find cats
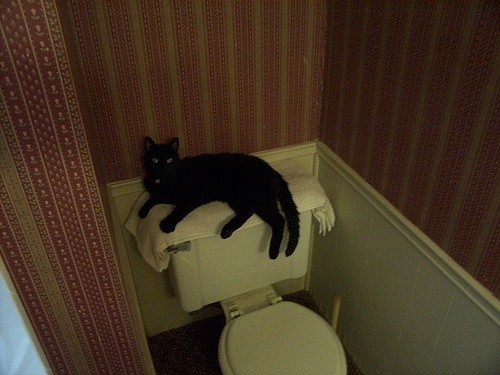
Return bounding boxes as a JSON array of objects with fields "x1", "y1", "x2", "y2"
[{"x1": 138, "y1": 136, "x2": 301, "y2": 260}]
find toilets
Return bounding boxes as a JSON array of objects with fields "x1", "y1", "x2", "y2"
[{"x1": 167, "y1": 160, "x2": 347, "y2": 374}]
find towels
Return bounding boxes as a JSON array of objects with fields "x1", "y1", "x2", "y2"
[{"x1": 123, "y1": 158, "x2": 335, "y2": 272}]
